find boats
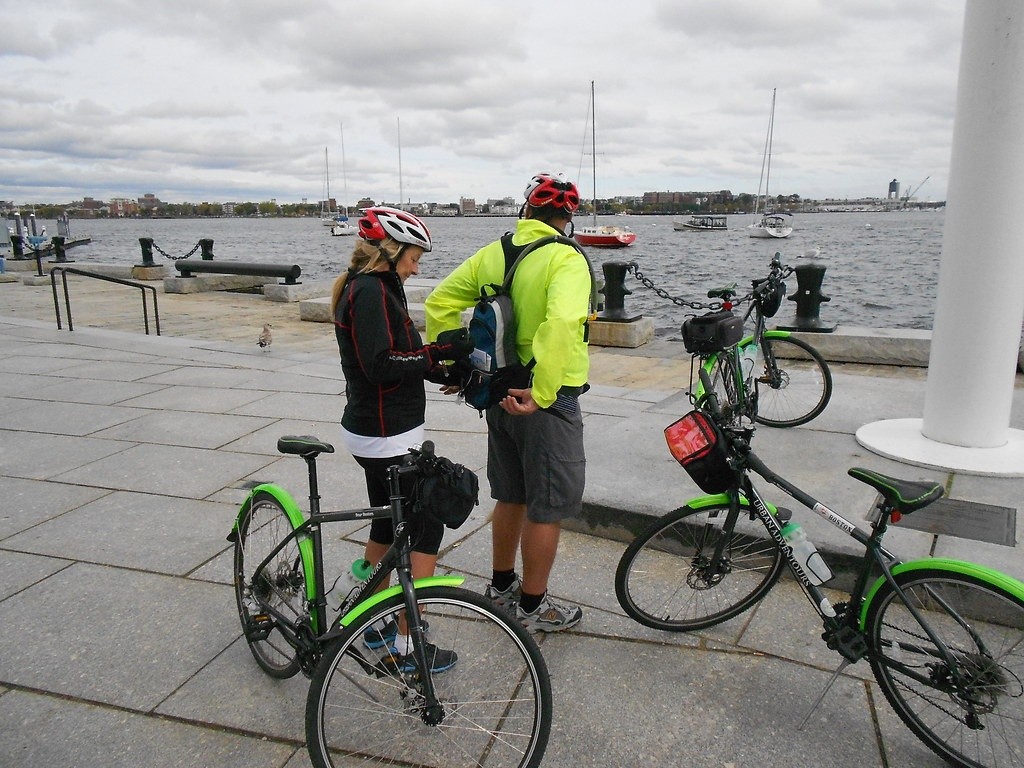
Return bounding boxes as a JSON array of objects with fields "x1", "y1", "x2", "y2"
[{"x1": 672, "y1": 214, "x2": 729, "y2": 232}]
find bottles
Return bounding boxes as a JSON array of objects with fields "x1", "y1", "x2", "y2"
[
  {"x1": 738, "y1": 346, "x2": 748, "y2": 381},
  {"x1": 781, "y1": 522, "x2": 832, "y2": 585},
  {"x1": 326, "y1": 559, "x2": 374, "y2": 610},
  {"x1": 745, "y1": 345, "x2": 758, "y2": 378}
]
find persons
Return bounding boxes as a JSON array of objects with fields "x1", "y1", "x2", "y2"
[
  {"x1": 424, "y1": 168, "x2": 591, "y2": 633},
  {"x1": 332, "y1": 206, "x2": 469, "y2": 673}
]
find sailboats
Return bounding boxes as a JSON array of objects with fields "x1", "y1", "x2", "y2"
[
  {"x1": 746, "y1": 86, "x2": 797, "y2": 239},
  {"x1": 570, "y1": 79, "x2": 637, "y2": 248},
  {"x1": 328, "y1": 121, "x2": 360, "y2": 237},
  {"x1": 320, "y1": 146, "x2": 341, "y2": 226}
]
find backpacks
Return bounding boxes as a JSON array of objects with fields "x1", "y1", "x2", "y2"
[{"x1": 459, "y1": 233, "x2": 597, "y2": 410}]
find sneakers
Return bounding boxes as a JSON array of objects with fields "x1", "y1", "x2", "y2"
[
  {"x1": 484, "y1": 574, "x2": 522, "y2": 608},
  {"x1": 389, "y1": 639, "x2": 457, "y2": 672},
  {"x1": 364, "y1": 612, "x2": 429, "y2": 648},
  {"x1": 515, "y1": 592, "x2": 582, "y2": 634}
]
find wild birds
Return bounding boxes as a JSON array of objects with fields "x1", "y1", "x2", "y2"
[
  {"x1": 254, "y1": 323, "x2": 274, "y2": 353},
  {"x1": 796, "y1": 247, "x2": 821, "y2": 264}
]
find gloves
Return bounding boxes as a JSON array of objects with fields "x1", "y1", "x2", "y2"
[
  {"x1": 440, "y1": 360, "x2": 472, "y2": 386},
  {"x1": 436, "y1": 328, "x2": 475, "y2": 360}
]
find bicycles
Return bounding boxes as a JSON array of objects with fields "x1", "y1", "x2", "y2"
[
  {"x1": 678, "y1": 251, "x2": 834, "y2": 429},
  {"x1": 226, "y1": 434, "x2": 556, "y2": 768},
  {"x1": 615, "y1": 364, "x2": 1024, "y2": 768}
]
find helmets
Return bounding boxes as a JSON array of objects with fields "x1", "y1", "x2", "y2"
[
  {"x1": 359, "y1": 206, "x2": 432, "y2": 253},
  {"x1": 525, "y1": 172, "x2": 580, "y2": 213}
]
